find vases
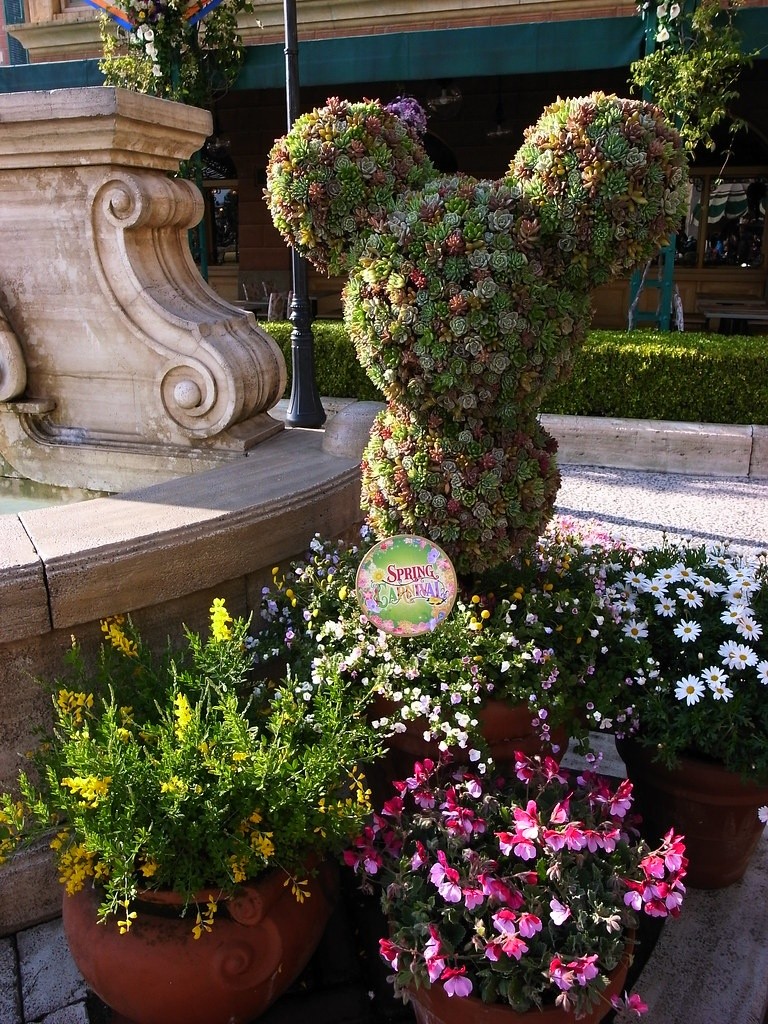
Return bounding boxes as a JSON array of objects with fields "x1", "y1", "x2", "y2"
[
  {"x1": 59, "y1": 847, "x2": 337, "y2": 1024},
  {"x1": 387, "y1": 913, "x2": 634, "y2": 1024},
  {"x1": 363, "y1": 691, "x2": 574, "y2": 780},
  {"x1": 641, "y1": 740, "x2": 768, "y2": 890}
]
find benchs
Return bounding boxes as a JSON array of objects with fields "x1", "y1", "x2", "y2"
[{"x1": 694, "y1": 305, "x2": 768, "y2": 337}]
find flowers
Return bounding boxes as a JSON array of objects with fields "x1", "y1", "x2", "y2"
[
  {"x1": 578, "y1": 527, "x2": 768, "y2": 829},
  {"x1": 252, "y1": 522, "x2": 584, "y2": 755},
  {"x1": 0, "y1": 595, "x2": 394, "y2": 941},
  {"x1": 336, "y1": 727, "x2": 691, "y2": 1018}
]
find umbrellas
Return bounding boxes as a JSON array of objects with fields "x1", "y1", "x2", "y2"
[{"x1": 692, "y1": 182, "x2": 749, "y2": 224}]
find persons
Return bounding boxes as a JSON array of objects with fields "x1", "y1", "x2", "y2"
[
  {"x1": 676, "y1": 224, "x2": 697, "y2": 254},
  {"x1": 707, "y1": 232, "x2": 737, "y2": 261},
  {"x1": 751, "y1": 234, "x2": 760, "y2": 256}
]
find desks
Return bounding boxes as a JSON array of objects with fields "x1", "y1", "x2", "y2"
[{"x1": 698, "y1": 295, "x2": 765, "y2": 333}]
